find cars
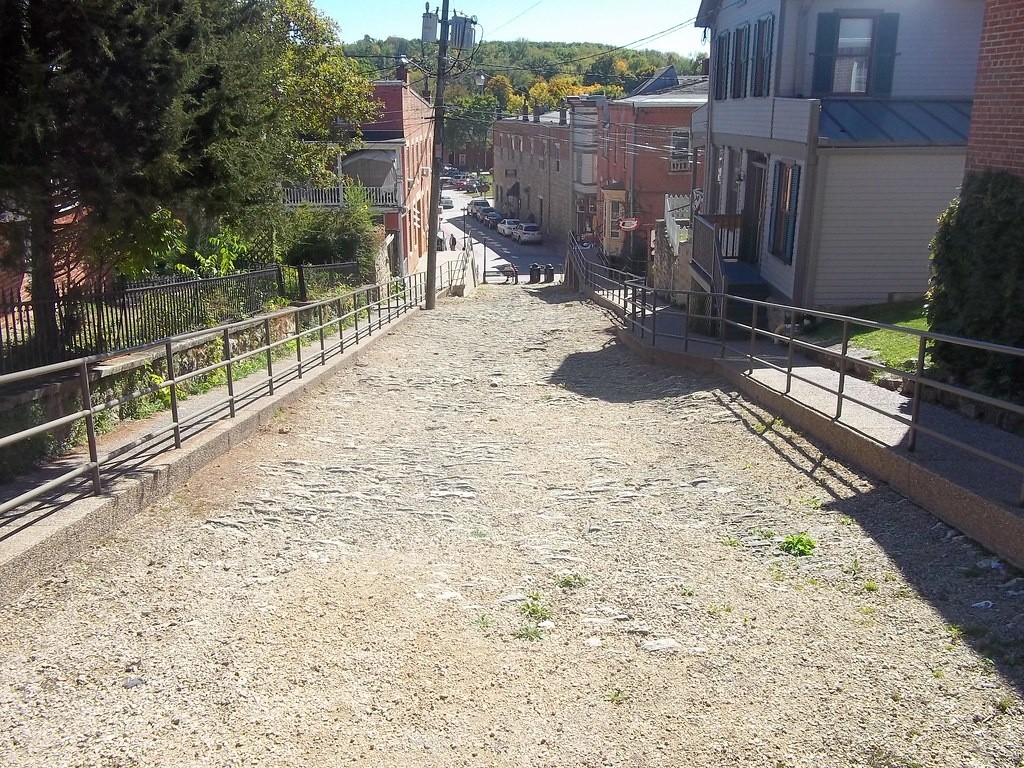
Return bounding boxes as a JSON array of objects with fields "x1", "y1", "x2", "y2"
[
  {"x1": 439, "y1": 195, "x2": 454, "y2": 209},
  {"x1": 453, "y1": 175, "x2": 469, "y2": 184},
  {"x1": 397, "y1": 53, "x2": 486, "y2": 311},
  {"x1": 484, "y1": 212, "x2": 502, "y2": 229},
  {"x1": 511, "y1": 223, "x2": 543, "y2": 245},
  {"x1": 476, "y1": 206, "x2": 495, "y2": 222},
  {"x1": 497, "y1": 219, "x2": 522, "y2": 238},
  {"x1": 466, "y1": 199, "x2": 490, "y2": 217}
]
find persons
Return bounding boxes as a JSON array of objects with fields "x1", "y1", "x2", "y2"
[
  {"x1": 527, "y1": 213, "x2": 534, "y2": 223},
  {"x1": 504, "y1": 263, "x2": 514, "y2": 282},
  {"x1": 503, "y1": 203, "x2": 507, "y2": 213},
  {"x1": 449, "y1": 234, "x2": 456, "y2": 251}
]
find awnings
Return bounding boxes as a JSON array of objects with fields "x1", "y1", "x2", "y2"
[{"x1": 506, "y1": 182, "x2": 519, "y2": 197}]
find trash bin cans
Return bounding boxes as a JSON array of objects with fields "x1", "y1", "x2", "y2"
[
  {"x1": 544, "y1": 263, "x2": 554, "y2": 282},
  {"x1": 529, "y1": 262, "x2": 541, "y2": 282}
]
